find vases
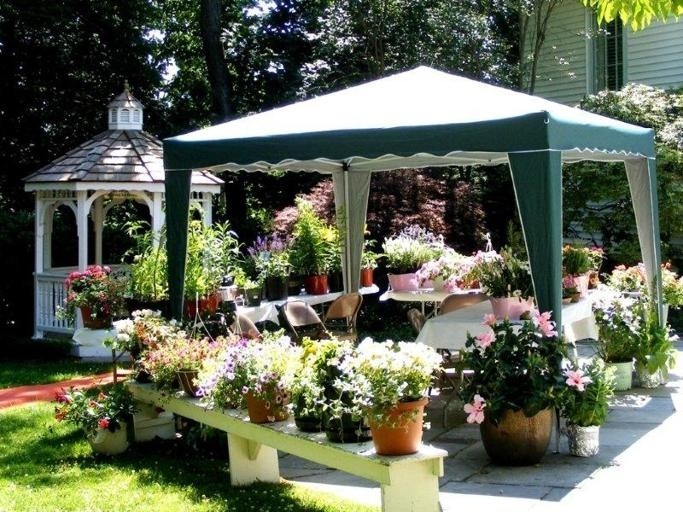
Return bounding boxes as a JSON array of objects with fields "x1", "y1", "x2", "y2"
[
  {"x1": 82, "y1": 419, "x2": 128, "y2": 456},
  {"x1": 478, "y1": 398, "x2": 599, "y2": 465},
  {"x1": 365, "y1": 392, "x2": 429, "y2": 455}
]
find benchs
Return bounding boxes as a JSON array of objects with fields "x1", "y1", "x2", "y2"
[{"x1": 122, "y1": 379, "x2": 446, "y2": 511}]
